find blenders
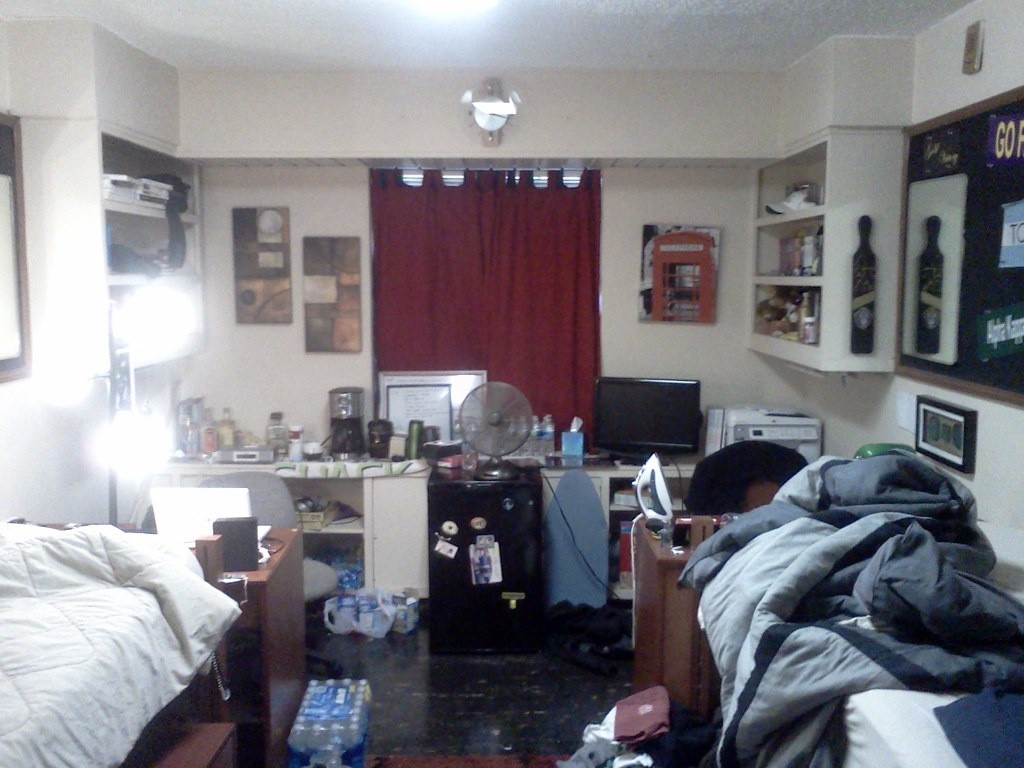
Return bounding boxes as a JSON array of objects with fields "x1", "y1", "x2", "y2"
[{"x1": 329, "y1": 386, "x2": 366, "y2": 460}]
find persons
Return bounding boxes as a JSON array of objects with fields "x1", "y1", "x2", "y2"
[{"x1": 472, "y1": 549, "x2": 492, "y2": 584}]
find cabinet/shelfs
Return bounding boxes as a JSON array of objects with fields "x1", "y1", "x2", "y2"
[
  {"x1": 747, "y1": 127, "x2": 905, "y2": 377},
  {"x1": 103, "y1": 139, "x2": 207, "y2": 359},
  {"x1": 292, "y1": 466, "x2": 429, "y2": 603},
  {"x1": 543, "y1": 477, "x2": 728, "y2": 603}
]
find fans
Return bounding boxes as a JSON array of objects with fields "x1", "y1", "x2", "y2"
[{"x1": 458, "y1": 379, "x2": 533, "y2": 476}]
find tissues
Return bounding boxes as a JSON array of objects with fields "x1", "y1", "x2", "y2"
[{"x1": 561, "y1": 415, "x2": 585, "y2": 456}]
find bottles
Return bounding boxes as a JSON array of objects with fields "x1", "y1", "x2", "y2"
[
  {"x1": 265, "y1": 412, "x2": 289, "y2": 461},
  {"x1": 198, "y1": 407, "x2": 218, "y2": 453},
  {"x1": 461, "y1": 415, "x2": 555, "y2": 470},
  {"x1": 217, "y1": 408, "x2": 237, "y2": 450},
  {"x1": 288, "y1": 677, "x2": 373, "y2": 768}
]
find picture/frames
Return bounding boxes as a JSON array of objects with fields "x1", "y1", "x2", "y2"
[
  {"x1": 386, "y1": 383, "x2": 451, "y2": 445},
  {"x1": 378, "y1": 369, "x2": 490, "y2": 429},
  {"x1": 909, "y1": 396, "x2": 978, "y2": 472}
]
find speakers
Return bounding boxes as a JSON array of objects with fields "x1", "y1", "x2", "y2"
[{"x1": 213, "y1": 518, "x2": 259, "y2": 572}]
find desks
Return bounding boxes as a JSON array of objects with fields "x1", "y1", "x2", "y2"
[
  {"x1": 46, "y1": 525, "x2": 307, "y2": 768},
  {"x1": 425, "y1": 464, "x2": 543, "y2": 652},
  {"x1": 630, "y1": 513, "x2": 724, "y2": 728}
]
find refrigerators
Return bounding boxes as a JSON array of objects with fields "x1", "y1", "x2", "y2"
[{"x1": 427, "y1": 464, "x2": 547, "y2": 655}]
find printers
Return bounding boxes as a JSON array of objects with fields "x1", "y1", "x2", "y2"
[{"x1": 705, "y1": 406, "x2": 822, "y2": 467}]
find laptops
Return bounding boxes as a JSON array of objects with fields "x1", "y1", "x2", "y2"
[{"x1": 148, "y1": 484, "x2": 271, "y2": 549}]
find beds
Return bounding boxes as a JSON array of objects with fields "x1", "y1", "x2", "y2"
[
  {"x1": 675, "y1": 477, "x2": 1024, "y2": 768},
  {"x1": 0, "y1": 513, "x2": 249, "y2": 768}
]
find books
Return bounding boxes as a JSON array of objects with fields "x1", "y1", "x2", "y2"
[{"x1": 103, "y1": 173, "x2": 174, "y2": 210}]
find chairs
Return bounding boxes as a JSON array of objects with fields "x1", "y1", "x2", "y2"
[
  {"x1": 685, "y1": 440, "x2": 810, "y2": 520},
  {"x1": 201, "y1": 470, "x2": 344, "y2": 680}
]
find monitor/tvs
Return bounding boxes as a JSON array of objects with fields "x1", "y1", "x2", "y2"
[{"x1": 592, "y1": 376, "x2": 700, "y2": 466}]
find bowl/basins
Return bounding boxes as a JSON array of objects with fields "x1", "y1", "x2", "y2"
[{"x1": 303, "y1": 441, "x2": 327, "y2": 459}]
